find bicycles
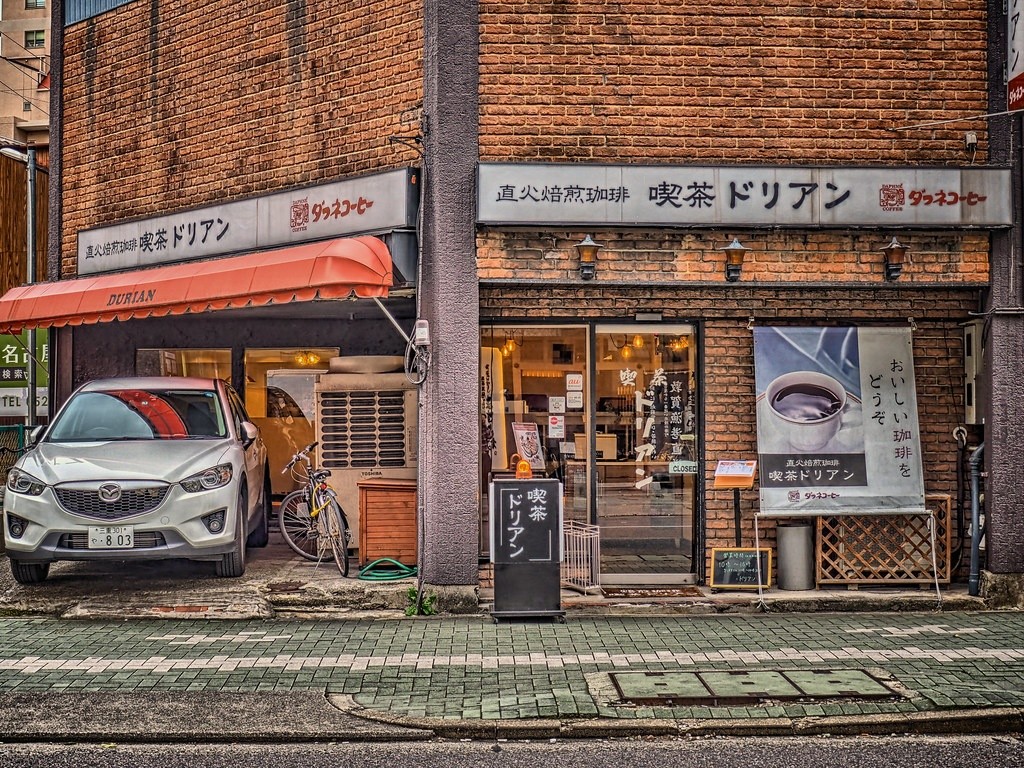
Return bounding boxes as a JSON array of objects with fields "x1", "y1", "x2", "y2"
[{"x1": 278, "y1": 441, "x2": 352, "y2": 578}]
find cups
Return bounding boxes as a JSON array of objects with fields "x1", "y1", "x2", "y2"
[{"x1": 765, "y1": 371, "x2": 848, "y2": 452}]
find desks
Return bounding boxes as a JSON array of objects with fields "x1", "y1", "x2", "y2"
[
  {"x1": 566, "y1": 461, "x2": 684, "y2": 518},
  {"x1": 523, "y1": 412, "x2": 637, "y2": 461}
]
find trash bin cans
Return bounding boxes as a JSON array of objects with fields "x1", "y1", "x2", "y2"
[{"x1": 777, "y1": 522, "x2": 814, "y2": 591}]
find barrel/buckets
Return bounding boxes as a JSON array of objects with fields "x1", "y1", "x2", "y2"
[{"x1": 775, "y1": 525, "x2": 815, "y2": 591}]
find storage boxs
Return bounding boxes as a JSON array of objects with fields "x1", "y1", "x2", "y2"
[
  {"x1": 622, "y1": 334, "x2": 632, "y2": 358},
  {"x1": 574, "y1": 432, "x2": 618, "y2": 460},
  {"x1": 633, "y1": 333, "x2": 644, "y2": 348}
]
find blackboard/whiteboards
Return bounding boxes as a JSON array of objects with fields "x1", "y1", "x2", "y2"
[
  {"x1": 491, "y1": 479, "x2": 566, "y2": 623},
  {"x1": 710, "y1": 548, "x2": 772, "y2": 588}
]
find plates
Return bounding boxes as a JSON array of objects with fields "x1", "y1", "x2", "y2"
[{"x1": 756, "y1": 387, "x2": 862, "y2": 453}]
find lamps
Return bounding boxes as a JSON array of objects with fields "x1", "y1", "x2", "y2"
[
  {"x1": 295, "y1": 350, "x2": 321, "y2": 367},
  {"x1": 879, "y1": 236, "x2": 911, "y2": 282},
  {"x1": 501, "y1": 328, "x2": 516, "y2": 356},
  {"x1": 572, "y1": 234, "x2": 604, "y2": 281},
  {"x1": 720, "y1": 235, "x2": 752, "y2": 283}
]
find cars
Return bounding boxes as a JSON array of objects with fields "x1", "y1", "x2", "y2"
[{"x1": 2, "y1": 376, "x2": 272, "y2": 585}]
